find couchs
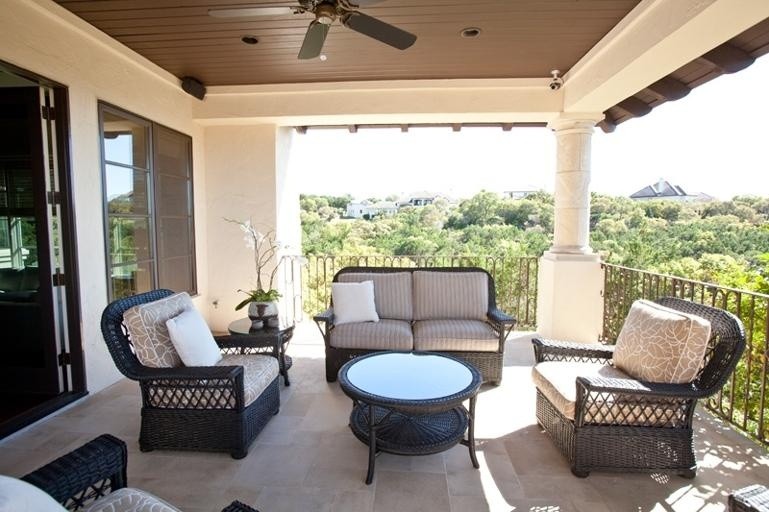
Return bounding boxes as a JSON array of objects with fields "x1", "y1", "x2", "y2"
[
  {"x1": 312, "y1": 266, "x2": 517, "y2": 387},
  {"x1": 531, "y1": 296, "x2": 748, "y2": 480}
]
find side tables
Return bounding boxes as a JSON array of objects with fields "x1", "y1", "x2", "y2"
[{"x1": 228, "y1": 315, "x2": 296, "y2": 386}]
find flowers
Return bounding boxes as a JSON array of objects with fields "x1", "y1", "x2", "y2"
[{"x1": 220, "y1": 217, "x2": 299, "y2": 311}]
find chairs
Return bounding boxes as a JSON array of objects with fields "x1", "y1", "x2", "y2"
[
  {"x1": 0, "y1": 434, "x2": 260, "y2": 512},
  {"x1": 99, "y1": 288, "x2": 281, "y2": 459}
]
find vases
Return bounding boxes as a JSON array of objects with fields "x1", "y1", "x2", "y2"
[{"x1": 247, "y1": 302, "x2": 277, "y2": 321}]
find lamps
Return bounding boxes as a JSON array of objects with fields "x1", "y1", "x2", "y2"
[{"x1": 315, "y1": 3, "x2": 335, "y2": 25}]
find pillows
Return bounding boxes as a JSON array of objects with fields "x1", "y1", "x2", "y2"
[
  {"x1": 1, "y1": 475, "x2": 68, "y2": 512},
  {"x1": 165, "y1": 308, "x2": 223, "y2": 385},
  {"x1": 329, "y1": 280, "x2": 379, "y2": 328}
]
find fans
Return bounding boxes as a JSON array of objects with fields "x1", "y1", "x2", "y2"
[{"x1": 209, "y1": 0, "x2": 418, "y2": 61}]
